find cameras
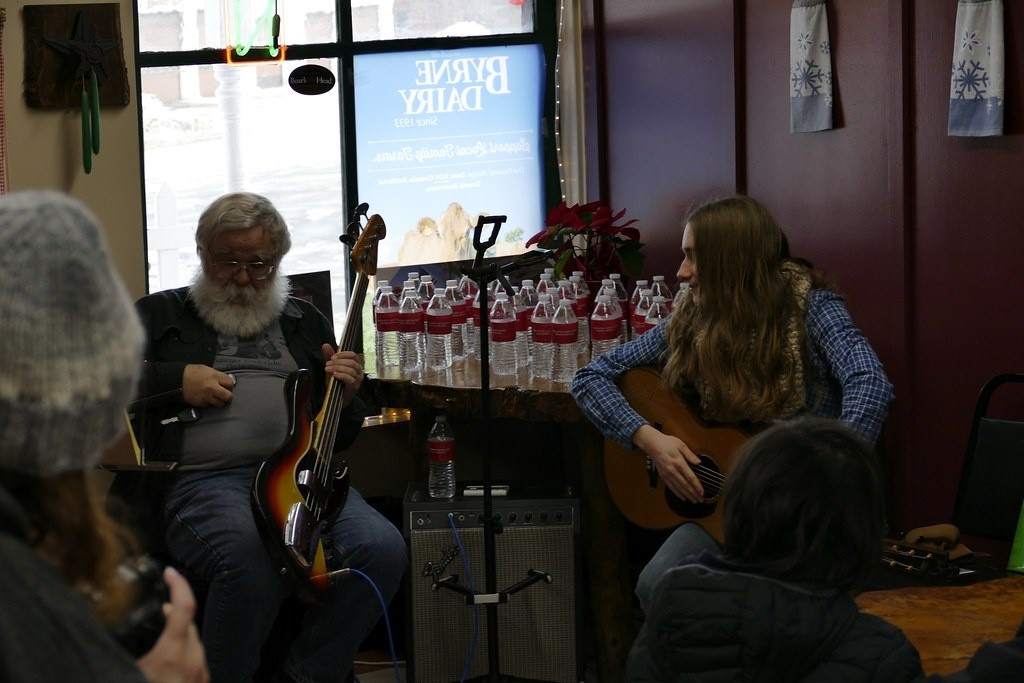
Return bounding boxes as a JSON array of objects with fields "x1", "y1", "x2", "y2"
[{"x1": 78, "y1": 553, "x2": 172, "y2": 661}]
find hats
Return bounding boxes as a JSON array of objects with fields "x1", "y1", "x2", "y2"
[{"x1": 2, "y1": 187, "x2": 148, "y2": 480}]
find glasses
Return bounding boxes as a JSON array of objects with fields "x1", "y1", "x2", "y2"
[{"x1": 205, "y1": 247, "x2": 279, "y2": 283}]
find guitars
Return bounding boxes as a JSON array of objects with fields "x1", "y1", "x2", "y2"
[
  {"x1": 604, "y1": 367, "x2": 1000, "y2": 588},
  {"x1": 254, "y1": 202, "x2": 387, "y2": 609}
]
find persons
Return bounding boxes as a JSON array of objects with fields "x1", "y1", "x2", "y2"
[
  {"x1": 570, "y1": 197, "x2": 895, "y2": 616},
  {"x1": 622, "y1": 421, "x2": 1023, "y2": 683},
  {"x1": 130, "y1": 193, "x2": 407, "y2": 683},
  {"x1": 1, "y1": 189, "x2": 207, "y2": 683}
]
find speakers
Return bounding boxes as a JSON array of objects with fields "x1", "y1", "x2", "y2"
[{"x1": 404, "y1": 412, "x2": 580, "y2": 683}]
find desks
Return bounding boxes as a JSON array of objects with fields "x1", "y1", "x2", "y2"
[{"x1": 347, "y1": 351, "x2": 632, "y2": 683}]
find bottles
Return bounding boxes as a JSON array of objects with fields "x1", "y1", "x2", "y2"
[
  {"x1": 425, "y1": 415, "x2": 456, "y2": 501},
  {"x1": 373, "y1": 266, "x2": 689, "y2": 385}
]
